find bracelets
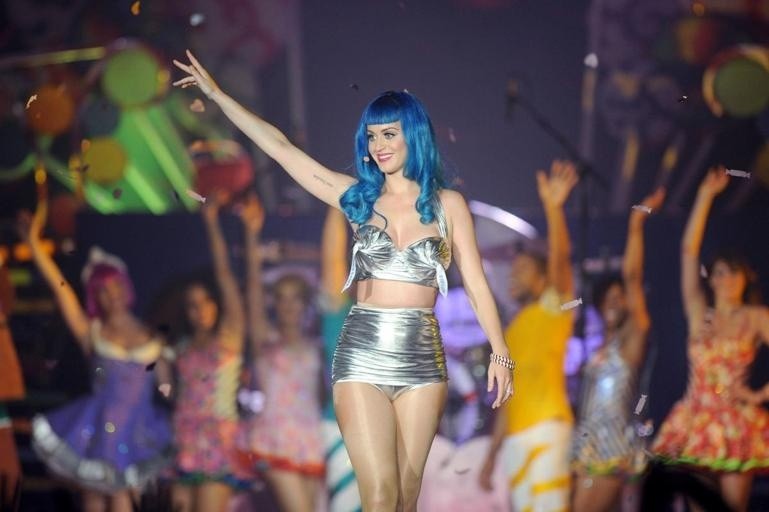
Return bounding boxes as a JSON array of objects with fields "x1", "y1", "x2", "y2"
[{"x1": 489, "y1": 353, "x2": 514, "y2": 371}]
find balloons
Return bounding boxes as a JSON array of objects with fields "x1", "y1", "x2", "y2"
[
  {"x1": 27, "y1": 88, "x2": 74, "y2": 134},
  {"x1": 85, "y1": 141, "x2": 126, "y2": 186},
  {"x1": 702, "y1": 53, "x2": 768, "y2": 113},
  {"x1": 99, "y1": 49, "x2": 159, "y2": 104}
]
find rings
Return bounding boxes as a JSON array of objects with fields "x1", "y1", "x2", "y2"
[{"x1": 506, "y1": 391, "x2": 513, "y2": 394}]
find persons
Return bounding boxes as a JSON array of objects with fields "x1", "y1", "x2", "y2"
[
  {"x1": 655, "y1": 164, "x2": 769, "y2": 512},
  {"x1": 573, "y1": 181, "x2": 669, "y2": 512},
  {"x1": 235, "y1": 193, "x2": 332, "y2": 512},
  {"x1": 173, "y1": 49, "x2": 514, "y2": 512},
  {"x1": 30, "y1": 165, "x2": 168, "y2": 511},
  {"x1": 170, "y1": 184, "x2": 246, "y2": 510},
  {"x1": 499, "y1": 153, "x2": 580, "y2": 511}
]
